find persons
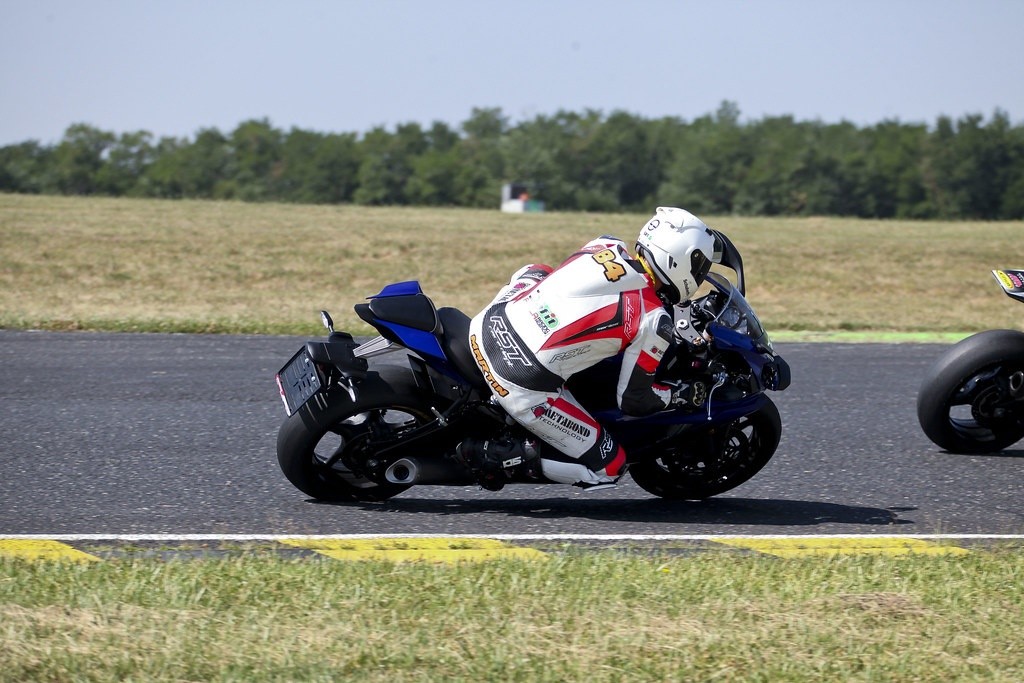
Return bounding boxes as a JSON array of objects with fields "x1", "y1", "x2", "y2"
[{"x1": 456, "y1": 207, "x2": 712, "y2": 491}]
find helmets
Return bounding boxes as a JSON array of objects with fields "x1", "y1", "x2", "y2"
[{"x1": 635, "y1": 206, "x2": 716, "y2": 307}]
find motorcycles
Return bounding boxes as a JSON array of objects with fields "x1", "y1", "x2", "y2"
[
  {"x1": 274, "y1": 231, "x2": 793, "y2": 507},
  {"x1": 916, "y1": 269, "x2": 1024, "y2": 455}
]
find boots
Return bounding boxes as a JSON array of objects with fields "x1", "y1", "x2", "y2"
[{"x1": 456, "y1": 437, "x2": 549, "y2": 491}]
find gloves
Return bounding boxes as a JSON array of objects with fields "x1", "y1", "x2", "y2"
[{"x1": 661, "y1": 378, "x2": 707, "y2": 412}]
